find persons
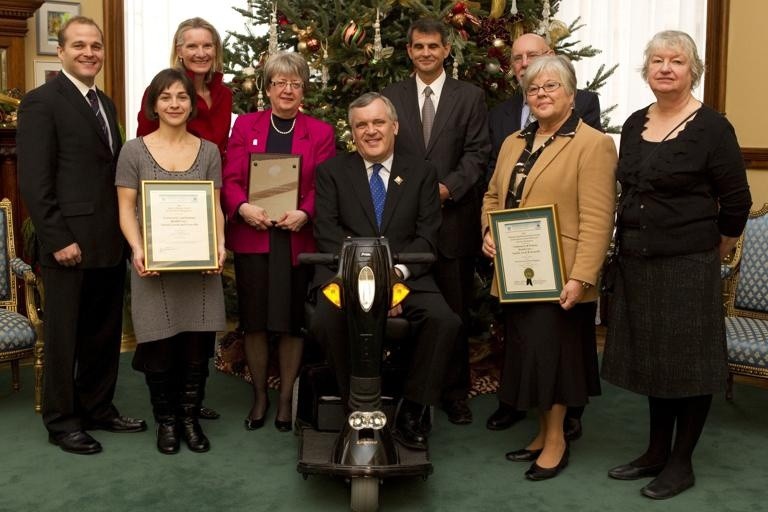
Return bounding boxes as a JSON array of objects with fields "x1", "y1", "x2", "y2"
[
  {"x1": 481, "y1": 54, "x2": 618, "y2": 481},
  {"x1": 303, "y1": 91, "x2": 461, "y2": 449},
  {"x1": 484, "y1": 33, "x2": 603, "y2": 440},
  {"x1": 381, "y1": 16, "x2": 492, "y2": 424},
  {"x1": 115, "y1": 69, "x2": 226, "y2": 454},
  {"x1": 219, "y1": 50, "x2": 337, "y2": 431},
  {"x1": 136, "y1": 17, "x2": 233, "y2": 419},
  {"x1": 599, "y1": 29, "x2": 754, "y2": 498},
  {"x1": 16, "y1": 15, "x2": 148, "y2": 454}
]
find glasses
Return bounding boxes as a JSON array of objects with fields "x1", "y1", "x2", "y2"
[
  {"x1": 525, "y1": 80, "x2": 563, "y2": 96},
  {"x1": 269, "y1": 80, "x2": 305, "y2": 89}
]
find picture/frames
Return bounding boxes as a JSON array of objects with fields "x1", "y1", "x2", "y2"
[
  {"x1": 36, "y1": 1, "x2": 81, "y2": 56},
  {"x1": 141, "y1": 180, "x2": 219, "y2": 272},
  {"x1": 487, "y1": 203, "x2": 565, "y2": 303},
  {"x1": 33, "y1": 60, "x2": 64, "y2": 88}
]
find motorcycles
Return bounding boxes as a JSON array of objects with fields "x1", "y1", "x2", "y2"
[{"x1": 297, "y1": 235, "x2": 434, "y2": 512}]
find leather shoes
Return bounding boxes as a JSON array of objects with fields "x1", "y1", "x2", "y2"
[
  {"x1": 275, "y1": 410, "x2": 292, "y2": 432},
  {"x1": 110, "y1": 416, "x2": 146, "y2": 433},
  {"x1": 487, "y1": 406, "x2": 528, "y2": 430},
  {"x1": 525, "y1": 445, "x2": 569, "y2": 481},
  {"x1": 563, "y1": 417, "x2": 582, "y2": 441},
  {"x1": 158, "y1": 407, "x2": 220, "y2": 455},
  {"x1": 395, "y1": 413, "x2": 427, "y2": 449},
  {"x1": 506, "y1": 448, "x2": 543, "y2": 462},
  {"x1": 448, "y1": 403, "x2": 472, "y2": 425},
  {"x1": 245, "y1": 416, "x2": 264, "y2": 430},
  {"x1": 49, "y1": 430, "x2": 101, "y2": 454},
  {"x1": 640, "y1": 470, "x2": 695, "y2": 500},
  {"x1": 608, "y1": 458, "x2": 666, "y2": 480}
]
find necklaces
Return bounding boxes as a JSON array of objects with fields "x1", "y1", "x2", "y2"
[{"x1": 271, "y1": 112, "x2": 296, "y2": 135}]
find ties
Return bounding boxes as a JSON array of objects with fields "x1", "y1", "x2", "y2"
[
  {"x1": 369, "y1": 164, "x2": 386, "y2": 232},
  {"x1": 86, "y1": 90, "x2": 109, "y2": 146},
  {"x1": 422, "y1": 86, "x2": 435, "y2": 150}
]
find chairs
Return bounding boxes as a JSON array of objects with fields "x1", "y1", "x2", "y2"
[
  {"x1": 721, "y1": 203, "x2": 768, "y2": 403},
  {"x1": 0, "y1": 197, "x2": 45, "y2": 414}
]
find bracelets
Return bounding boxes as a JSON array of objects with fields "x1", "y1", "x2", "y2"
[{"x1": 582, "y1": 281, "x2": 592, "y2": 289}]
